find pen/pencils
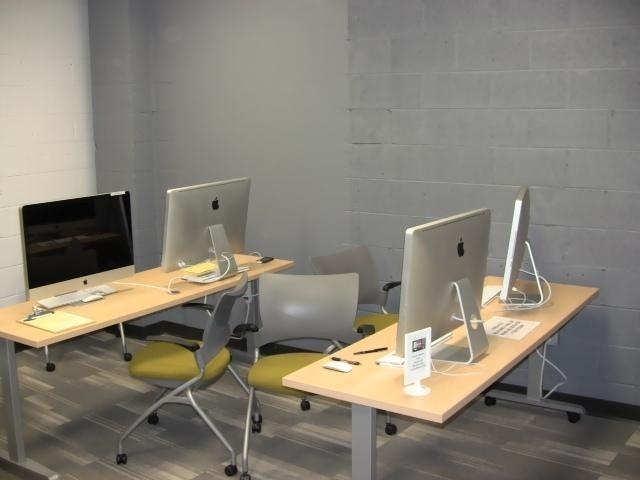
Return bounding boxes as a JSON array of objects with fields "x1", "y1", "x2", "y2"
[
  {"x1": 353, "y1": 347, "x2": 387, "y2": 355},
  {"x1": 332, "y1": 357, "x2": 360, "y2": 365}
]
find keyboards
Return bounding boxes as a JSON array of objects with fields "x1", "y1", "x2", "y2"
[
  {"x1": 36, "y1": 284, "x2": 120, "y2": 311},
  {"x1": 379, "y1": 331, "x2": 453, "y2": 366},
  {"x1": 187, "y1": 260, "x2": 254, "y2": 287},
  {"x1": 482, "y1": 285, "x2": 502, "y2": 307}
]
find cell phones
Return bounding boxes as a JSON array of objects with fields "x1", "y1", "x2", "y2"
[{"x1": 258, "y1": 257, "x2": 274, "y2": 263}]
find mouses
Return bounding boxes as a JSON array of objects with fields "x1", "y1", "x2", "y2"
[
  {"x1": 81, "y1": 292, "x2": 102, "y2": 303},
  {"x1": 321, "y1": 357, "x2": 352, "y2": 378}
]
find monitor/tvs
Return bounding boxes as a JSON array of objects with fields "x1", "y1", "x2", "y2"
[
  {"x1": 396, "y1": 208, "x2": 491, "y2": 365},
  {"x1": 18, "y1": 191, "x2": 135, "y2": 302},
  {"x1": 160, "y1": 176, "x2": 251, "y2": 284},
  {"x1": 500, "y1": 183, "x2": 543, "y2": 306}
]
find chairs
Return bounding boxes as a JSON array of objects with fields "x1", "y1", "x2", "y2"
[
  {"x1": 240, "y1": 270, "x2": 361, "y2": 480},
  {"x1": 116, "y1": 270, "x2": 262, "y2": 477},
  {"x1": 299, "y1": 279, "x2": 404, "y2": 438},
  {"x1": 43, "y1": 320, "x2": 132, "y2": 373}
]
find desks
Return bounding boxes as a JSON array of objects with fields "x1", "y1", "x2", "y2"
[
  {"x1": 0, "y1": 253, "x2": 296, "y2": 479},
  {"x1": 281, "y1": 274, "x2": 600, "y2": 479}
]
